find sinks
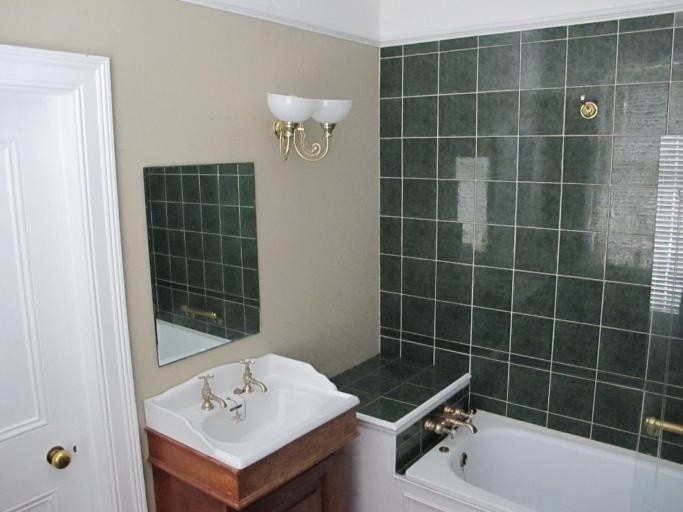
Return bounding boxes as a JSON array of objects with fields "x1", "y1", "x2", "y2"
[{"x1": 143, "y1": 353, "x2": 360, "y2": 469}]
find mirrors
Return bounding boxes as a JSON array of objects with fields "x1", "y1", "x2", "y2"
[{"x1": 142, "y1": 160, "x2": 261, "y2": 369}]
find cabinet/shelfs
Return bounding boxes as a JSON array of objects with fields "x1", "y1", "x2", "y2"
[{"x1": 149, "y1": 454, "x2": 344, "y2": 512}]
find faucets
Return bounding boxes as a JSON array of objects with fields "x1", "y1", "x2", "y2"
[
  {"x1": 439, "y1": 412, "x2": 477, "y2": 435},
  {"x1": 233, "y1": 360, "x2": 266, "y2": 395},
  {"x1": 197, "y1": 375, "x2": 228, "y2": 412}
]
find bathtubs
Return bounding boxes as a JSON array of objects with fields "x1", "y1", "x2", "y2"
[
  {"x1": 155, "y1": 317, "x2": 230, "y2": 367},
  {"x1": 405, "y1": 409, "x2": 682, "y2": 511}
]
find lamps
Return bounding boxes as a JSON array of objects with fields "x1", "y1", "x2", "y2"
[{"x1": 265, "y1": 91, "x2": 352, "y2": 164}]
